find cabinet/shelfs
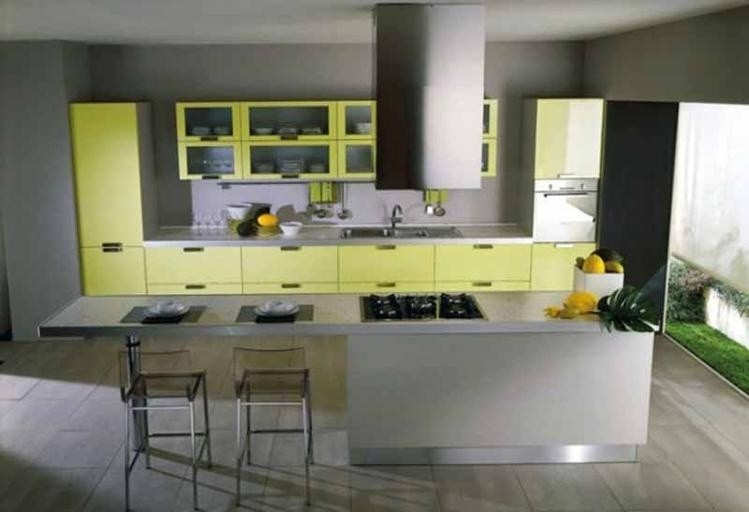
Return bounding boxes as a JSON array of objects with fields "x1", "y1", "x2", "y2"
[
  {"x1": 479, "y1": 97, "x2": 498, "y2": 179},
  {"x1": 533, "y1": 241, "x2": 598, "y2": 292},
  {"x1": 520, "y1": 98, "x2": 606, "y2": 181},
  {"x1": 177, "y1": 99, "x2": 240, "y2": 184},
  {"x1": 82, "y1": 246, "x2": 148, "y2": 297},
  {"x1": 143, "y1": 246, "x2": 532, "y2": 295},
  {"x1": 339, "y1": 99, "x2": 377, "y2": 182},
  {"x1": 68, "y1": 100, "x2": 160, "y2": 247},
  {"x1": 242, "y1": 100, "x2": 338, "y2": 183}
]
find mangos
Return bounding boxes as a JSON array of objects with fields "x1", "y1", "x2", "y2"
[
  {"x1": 562, "y1": 291, "x2": 598, "y2": 313},
  {"x1": 605, "y1": 261, "x2": 624, "y2": 273},
  {"x1": 582, "y1": 254, "x2": 606, "y2": 274}
]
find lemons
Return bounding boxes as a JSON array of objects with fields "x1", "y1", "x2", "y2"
[{"x1": 257, "y1": 214, "x2": 278, "y2": 226}]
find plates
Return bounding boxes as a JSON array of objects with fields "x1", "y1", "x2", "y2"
[
  {"x1": 254, "y1": 304, "x2": 300, "y2": 317},
  {"x1": 144, "y1": 304, "x2": 190, "y2": 318}
]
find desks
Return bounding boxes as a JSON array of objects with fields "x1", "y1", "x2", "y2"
[{"x1": 36, "y1": 294, "x2": 364, "y2": 455}]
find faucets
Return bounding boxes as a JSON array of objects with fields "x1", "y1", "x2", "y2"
[{"x1": 390, "y1": 203, "x2": 404, "y2": 228}]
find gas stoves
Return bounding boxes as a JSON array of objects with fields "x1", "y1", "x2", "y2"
[{"x1": 360, "y1": 293, "x2": 485, "y2": 321}]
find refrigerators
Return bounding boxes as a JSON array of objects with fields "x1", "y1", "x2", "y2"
[{"x1": 523, "y1": 98, "x2": 605, "y2": 293}]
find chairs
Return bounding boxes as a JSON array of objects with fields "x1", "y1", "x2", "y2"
[{"x1": 114, "y1": 344, "x2": 319, "y2": 509}]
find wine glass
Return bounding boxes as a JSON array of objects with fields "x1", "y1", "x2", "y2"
[{"x1": 191, "y1": 213, "x2": 229, "y2": 237}]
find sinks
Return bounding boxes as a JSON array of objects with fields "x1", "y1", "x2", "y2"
[
  {"x1": 338, "y1": 226, "x2": 394, "y2": 240},
  {"x1": 424, "y1": 225, "x2": 465, "y2": 238},
  {"x1": 391, "y1": 226, "x2": 429, "y2": 239}
]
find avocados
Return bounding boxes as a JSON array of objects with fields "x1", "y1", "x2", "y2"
[
  {"x1": 237, "y1": 219, "x2": 253, "y2": 236},
  {"x1": 254, "y1": 207, "x2": 269, "y2": 222}
]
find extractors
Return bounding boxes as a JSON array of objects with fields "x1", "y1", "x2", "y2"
[{"x1": 373, "y1": 2, "x2": 489, "y2": 191}]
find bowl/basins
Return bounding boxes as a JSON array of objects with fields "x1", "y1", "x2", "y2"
[
  {"x1": 254, "y1": 128, "x2": 274, "y2": 135},
  {"x1": 279, "y1": 222, "x2": 303, "y2": 235},
  {"x1": 226, "y1": 203, "x2": 252, "y2": 220}
]
